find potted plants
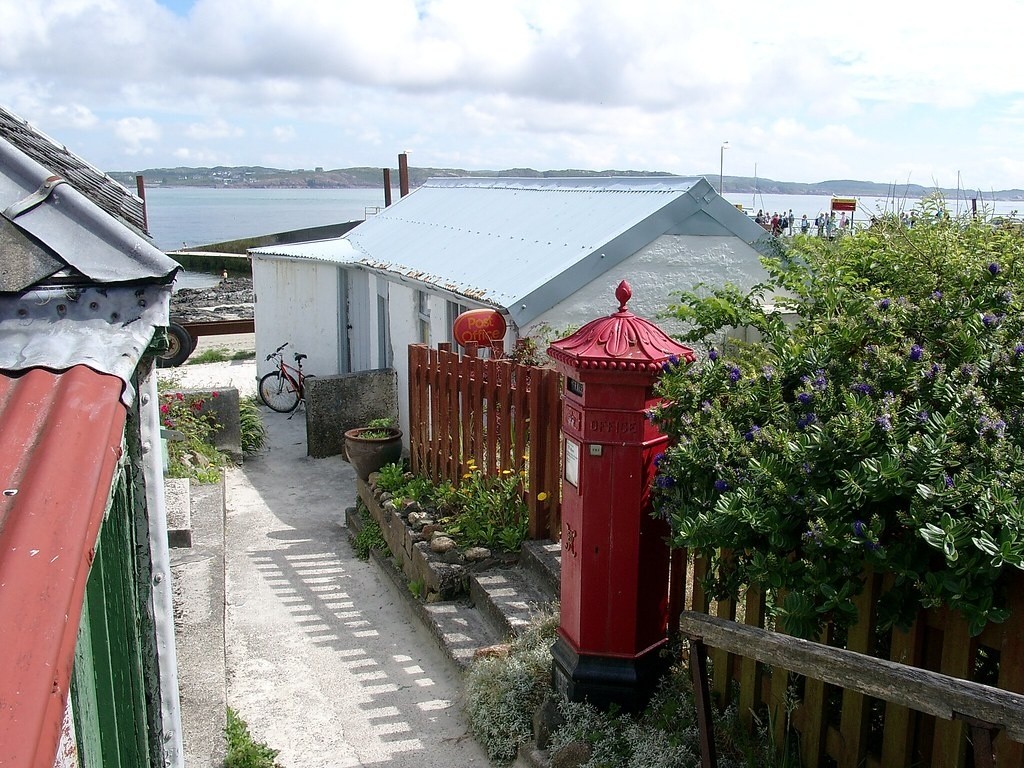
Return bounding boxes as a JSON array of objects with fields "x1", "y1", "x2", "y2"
[{"x1": 344, "y1": 415, "x2": 404, "y2": 481}]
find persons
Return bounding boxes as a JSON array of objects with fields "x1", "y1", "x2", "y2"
[
  {"x1": 756, "y1": 209, "x2": 847, "y2": 242},
  {"x1": 868, "y1": 208, "x2": 943, "y2": 232}
]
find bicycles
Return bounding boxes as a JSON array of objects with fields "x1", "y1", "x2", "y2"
[{"x1": 257, "y1": 343, "x2": 318, "y2": 419}]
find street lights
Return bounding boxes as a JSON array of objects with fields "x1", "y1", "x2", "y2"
[{"x1": 720, "y1": 141, "x2": 730, "y2": 196}]
